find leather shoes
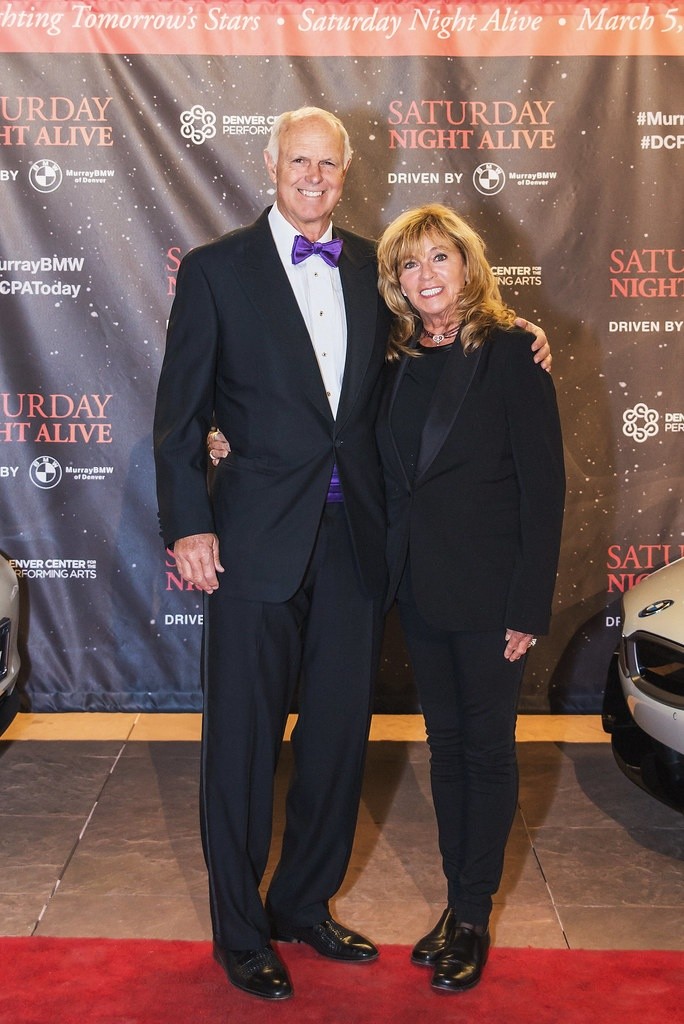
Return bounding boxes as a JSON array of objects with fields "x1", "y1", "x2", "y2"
[
  {"x1": 410, "y1": 910, "x2": 460, "y2": 966},
  {"x1": 212, "y1": 937, "x2": 292, "y2": 1001},
  {"x1": 269, "y1": 910, "x2": 379, "y2": 962},
  {"x1": 432, "y1": 920, "x2": 489, "y2": 991}
]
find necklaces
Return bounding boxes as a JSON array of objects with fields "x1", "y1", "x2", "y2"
[{"x1": 421, "y1": 317, "x2": 466, "y2": 344}]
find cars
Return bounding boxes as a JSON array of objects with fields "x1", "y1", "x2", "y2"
[{"x1": 613, "y1": 557, "x2": 684, "y2": 797}]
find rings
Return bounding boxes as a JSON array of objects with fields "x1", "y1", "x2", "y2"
[
  {"x1": 529, "y1": 638, "x2": 537, "y2": 647},
  {"x1": 210, "y1": 449, "x2": 217, "y2": 460},
  {"x1": 211, "y1": 431, "x2": 219, "y2": 442}
]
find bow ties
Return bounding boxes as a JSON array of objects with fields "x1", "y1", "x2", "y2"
[{"x1": 292, "y1": 235, "x2": 345, "y2": 267}]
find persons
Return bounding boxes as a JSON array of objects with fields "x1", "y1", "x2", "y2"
[{"x1": 154, "y1": 105, "x2": 566, "y2": 1000}]
